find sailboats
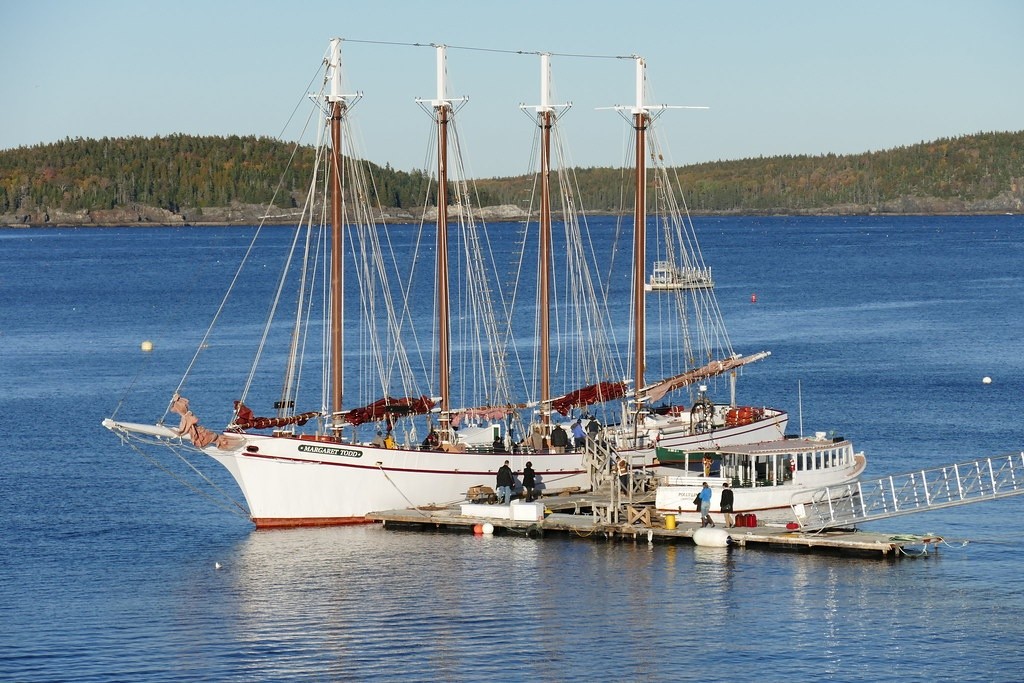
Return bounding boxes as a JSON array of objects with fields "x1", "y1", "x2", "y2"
[{"x1": 97, "y1": 42, "x2": 788, "y2": 529}]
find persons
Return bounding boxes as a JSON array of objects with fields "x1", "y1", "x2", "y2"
[
  {"x1": 493, "y1": 436, "x2": 504, "y2": 452},
  {"x1": 613, "y1": 456, "x2": 628, "y2": 497},
  {"x1": 570, "y1": 419, "x2": 586, "y2": 454},
  {"x1": 372, "y1": 431, "x2": 397, "y2": 449},
  {"x1": 531, "y1": 427, "x2": 543, "y2": 454},
  {"x1": 719, "y1": 483, "x2": 735, "y2": 528},
  {"x1": 585, "y1": 415, "x2": 603, "y2": 453},
  {"x1": 551, "y1": 423, "x2": 568, "y2": 454},
  {"x1": 496, "y1": 461, "x2": 514, "y2": 506},
  {"x1": 522, "y1": 462, "x2": 536, "y2": 502},
  {"x1": 699, "y1": 482, "x2": 715, "y2": 528},
  {"x1": 422, "y1": 434, "x2": 432, "y2": 450}
]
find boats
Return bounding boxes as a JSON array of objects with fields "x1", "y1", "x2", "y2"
[{"x1": 655, "y1": 432, "x2": 866, "y2": 513}]
